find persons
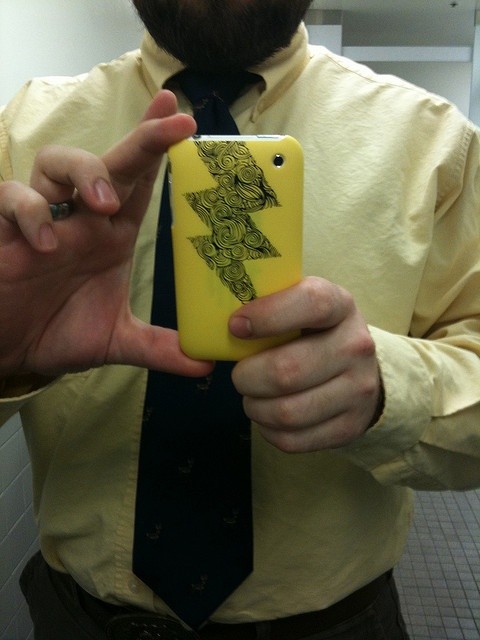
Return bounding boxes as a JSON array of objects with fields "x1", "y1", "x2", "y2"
[{"x1": 0, "y1": 3, "x2": 479, "y2": 638}]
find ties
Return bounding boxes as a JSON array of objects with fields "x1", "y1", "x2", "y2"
[{"x1": 131, "y1": 76, "x2": 262, "y2": 627}]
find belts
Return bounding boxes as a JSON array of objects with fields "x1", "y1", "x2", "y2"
[{"x1": 55, "y1": 571, "x2": 393, "y2": 640}]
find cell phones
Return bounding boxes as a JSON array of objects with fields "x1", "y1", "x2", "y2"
[{"x1": 167, "y1": 134, "x2": 304, "y2": 362}]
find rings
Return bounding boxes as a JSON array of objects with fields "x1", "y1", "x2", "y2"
[{"x1": 49, "y1": 199, "x2": 77, "y2": 222}]
